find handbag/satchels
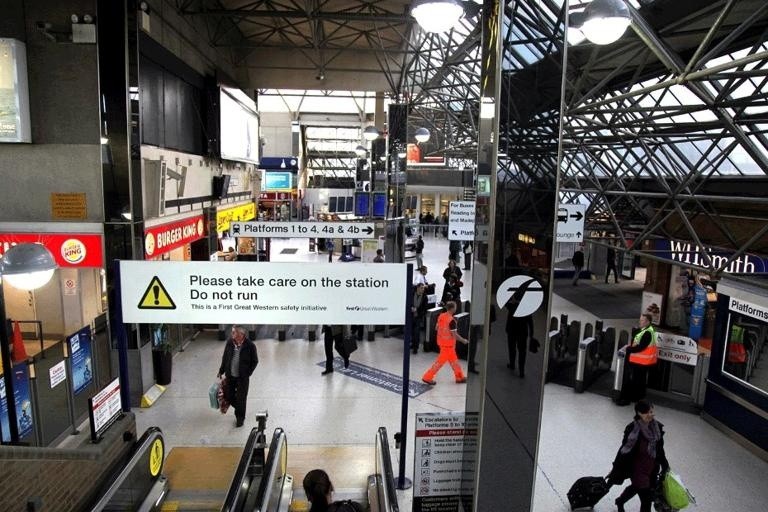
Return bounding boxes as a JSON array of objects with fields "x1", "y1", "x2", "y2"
[
  {"x1": 653, "y1": 470, "x2": 690, "y2": 511},
  {"x1": 208, "y1": 375, "x2": 230, "y2": 415}
]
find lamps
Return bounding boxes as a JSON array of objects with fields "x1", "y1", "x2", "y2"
[
  {"x1": 567, "y1": 11, "x2": 586, "y2": 47},
  {"x1": 414, "y1": 126, "x2": 430, "y2": 143},
  {"x1": 581, "y1": 0, "x2": 634, "y2": 47},
  {"x1": 399, "y1": 146, "x2": 406, "y2": 159},
  {"x1": 409, "y1": 0, "x2": 464, "y2": 33},
  {"x1": 356, "y1": 144, "x2": 369, "y2": 157},
  {"x1": 363, "y1": 125, "x2": 380, "y2": 141},
  {"x1": 379, "y1": 151, "x2": 386, "y2": 163}
]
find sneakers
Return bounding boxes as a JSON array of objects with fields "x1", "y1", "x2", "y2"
[
  {"x1": 344, "y1": 353, "x2": 350, "y2": 368},
  {"x1": 456, "y1": 376, "x2": 467, "y2": 383},
  {"x1": 421, "y1": 378, "x2": 437, "y2": 385},
  {"x1": 236, "y1": 415, "x2": 245, "y2": 428},
  {"x1": 615, "y1": 497, "x2": 625, "y2": 512},
  {"x1": 322, "y1": 368, "x2": 333, "y2": 374}
]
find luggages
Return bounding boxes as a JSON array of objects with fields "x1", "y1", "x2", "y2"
[{"x1": 566, "y1": 474, "x2": 610, "y2": 510}]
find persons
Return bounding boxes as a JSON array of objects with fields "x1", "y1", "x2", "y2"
[
  {"x1": 302, "y1": 469, "x2": 366, "y2": 512},
  {"x1": 605, "y1": 248, "x2": 619, "y2": 285},
  {"x1": 319, "y1": 212, "x2": 472, "y2": 384},
  {"x1": 726, "y1": 315, "x2": 754, "y2": 380},
  {"x1": 18, "y1": 409, "x2": 32, "y2": 431},
  {"x1": 217, "y1": 325, "x2": 259, "y2": 427},
  {"x1": 506, "y1": 310, "x2": 534, "y2": 379},
  {"x1": 603, "y1": 399, "x2": 670, "y2": 512},
  {"x1": 280, "y1": 201, "x2": 289, "y2": 222},
  {"x1": 571, "y1": 246, "x2": 586, "y2": 286},
  {"x1": 225, "y1": 247, "x2": 238, "y2": 261},
  {"x1": 625, "y1": 313, "x2": 657, "y2": 403},
  {"x1": 82, "y1": 364, "x2": 90, "y2": 380}
]
